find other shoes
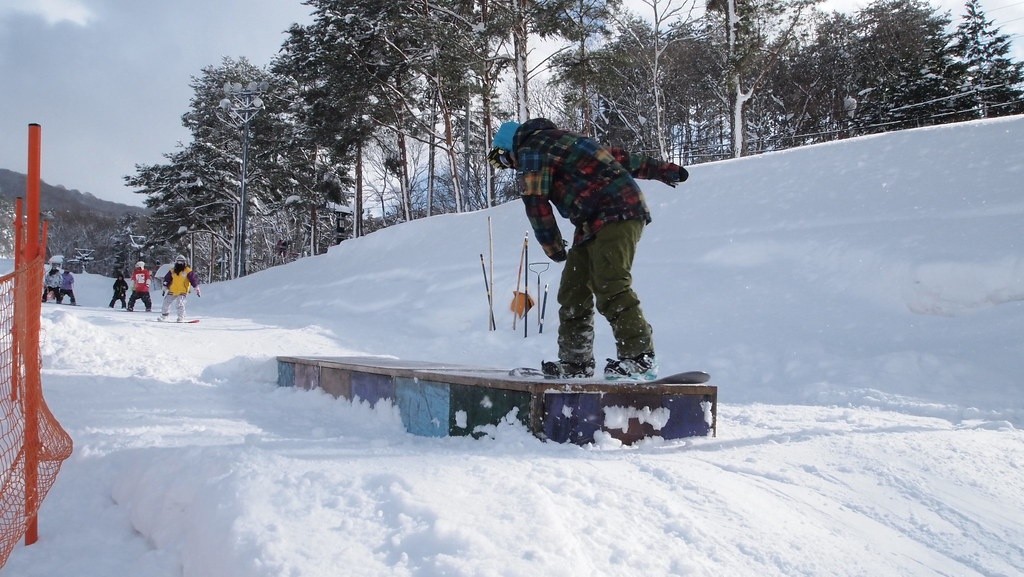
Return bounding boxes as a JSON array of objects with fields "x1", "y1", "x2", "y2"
[
  {"x1": 178, "y1": 317, "x2": 184, "y2": 322},
  {"x1": 42, "y1": 298, "x2": 46, "y2": 302},
  {"x1": 158, "y1": 315, "x2": 169, "y2": 321},
  {"x1": 147, "y1": 309, "x2": 151, "y2": 311},
  {"x1": 57, "y1": 300, "x2": 62, "y2": 303},
  {"x1": 72, "y1": 302, "x2": 76, "y2": 306},
  {"x1": 128, "y1": 307, "x2": 133, "y2": 310}
]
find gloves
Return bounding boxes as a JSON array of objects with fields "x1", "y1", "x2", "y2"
[
  {"x1": 194, "y1": 286, "x2": 200, "y2": 297},
  {"x1": 162, "y1": 287, "x2": 166, "y2": 296},
  {"x1": 542, "y1": 239, "x2": 567, "y2": 262},
  {"x1": 661, "y1": 161, "x2": 688, "y2": 188}
]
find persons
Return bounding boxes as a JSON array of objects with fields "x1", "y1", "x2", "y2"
[
  {"x1": 42, "y1": 264, "x2": 77, "y2": 306},
  {"x1": 157, "y1": 254, "x2": 201, "y2": 322},
  {"x1": 487, "y1": 119, "x2": 690, "y2": 382},
  {"x1": 109, "y1": 275, "x2": 128, "y2": 309},
  {"x1": 126, "y1": 261, "x2": 152, "y2": 311}
]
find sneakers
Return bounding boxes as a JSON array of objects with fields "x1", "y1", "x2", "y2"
[
  {"x1": 605, "y1": 359, "x2": 653, "y2": 375},
  {"x1": 543, "y1": 359, "x2": 595, "y2": 375}
]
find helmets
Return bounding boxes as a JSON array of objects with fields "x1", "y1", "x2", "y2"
[
  {"x1": 135, "y1": 261, "x2": 145, "y2": 267},
  {"x1": 65, "y1": 267, "x2": 70, "y2": 271},
  {"x1": 176, "y1": 254, "x2": 186, "y2": 260},
  {"x1": 118, "y1": 274, "x2": 123, "y2": 277}
]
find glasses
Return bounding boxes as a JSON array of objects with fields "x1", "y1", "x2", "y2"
[{"x1": 500, "y1": 154, "x2": 513, "y2": 168}]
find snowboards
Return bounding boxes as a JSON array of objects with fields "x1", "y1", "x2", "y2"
[
  {"x1": 146, "y1": 315, "x2": 204, "y2": 325},
  {"x1": 508, "y1": 366, "x2": 714, "y2": 384}
]
